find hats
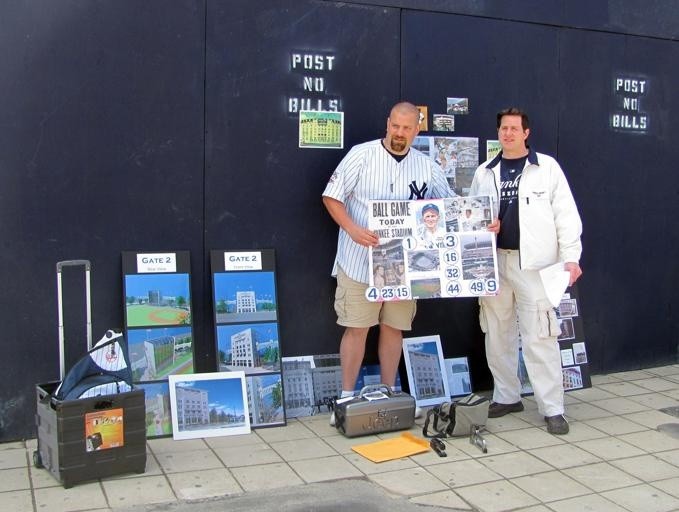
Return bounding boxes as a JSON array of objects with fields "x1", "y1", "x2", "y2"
[{"x1": 421, "y1": 203, "x2": 439, "y2": 216}]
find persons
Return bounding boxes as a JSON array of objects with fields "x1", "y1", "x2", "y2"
[
  {"x1": 373, "y1": 262, "x2": 385, "y2": 288},
  {"x1": 321, "y1": 101, "x2": 501, "y2": 424},
  {"x1": 396, "y1": 260, "x2": 406, "y2": 284},
  {"x1": 415, "y1": 202, "x2": 448, "y2": 250},
  {"x1": 463, "y1": 208, "x2": 475, "y2": 230},
  {"x1": 463, "y1": 108, "x2": 586, "y2": 433}
]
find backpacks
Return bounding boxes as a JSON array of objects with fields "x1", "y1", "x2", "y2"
[{"x1": 51, "y1": 327, "x2": 134, "y2": 401}]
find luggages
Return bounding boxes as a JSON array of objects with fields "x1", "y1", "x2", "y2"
[{"x1": 29, "y1": 258, "x2": 149, "y2": 488}]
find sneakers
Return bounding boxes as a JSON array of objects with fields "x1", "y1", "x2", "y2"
[
  {"x1": 415, "y1": 406, "x2": 423, "y2": 418},
  {"x1": 543, "y1": 413, "x2": 570, "y2": 436},
  {"x1": 329, "y1": 410, "x2": 338, "y2": 426},
  {"x1": 487, "y1": 398, "x2": 525, "y2": 418}
]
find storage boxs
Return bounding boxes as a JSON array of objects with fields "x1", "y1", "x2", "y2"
[{"x1": 28, "y1": 376, "x2": 149, "y2": 492}]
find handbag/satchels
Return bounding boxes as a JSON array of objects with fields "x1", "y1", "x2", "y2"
[{"x1": 431, "y1": 391, "x2": 490, "y2": 438}]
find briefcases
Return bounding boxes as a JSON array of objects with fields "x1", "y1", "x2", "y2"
[{"x1": 334, "y1": 383, "x2": 416, "y2": 438}]
